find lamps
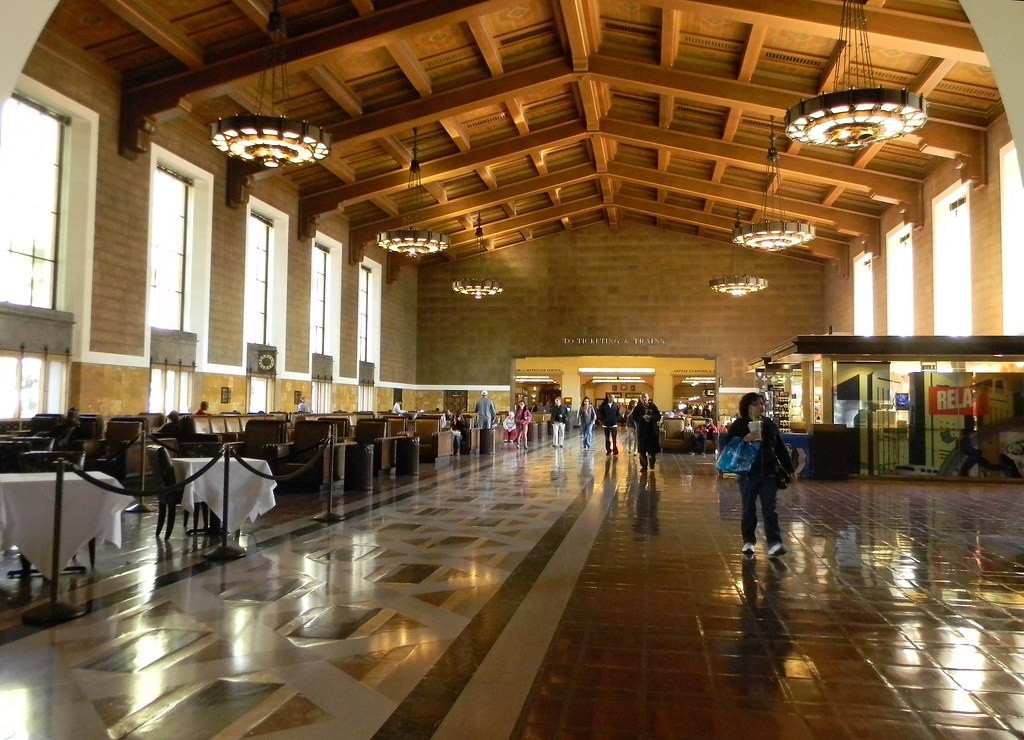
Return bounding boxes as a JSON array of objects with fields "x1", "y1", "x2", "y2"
[
  {"x1": 709, "y1": 203, "x2": 768, "y2": 296},
  {"x1": 784, "y1": 0, "x2": 928, "y2": 150},
  {"x1": 732, "y1": 119, "x2": 816, "y2": 251},
  {"x1": 210, "y1": 0, "x2": 332, "y2": 167},
  {"x1": 452, "y1": 210, "x2": 505, "y2": 299},
  {"x1": 377, "y1": 127, "x2": 451, "y2": 259}
]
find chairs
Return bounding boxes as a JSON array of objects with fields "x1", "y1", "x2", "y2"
[
  {"x1": 19, "y1": 451, "x2": 95, "y2": 569},
  {"x1": 146, "y1": 445, "x2": 208, "y2": 540}
]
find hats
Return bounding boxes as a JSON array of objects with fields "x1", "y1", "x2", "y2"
[{"x1": 480, "y1": 390, "x2": 488, "y2": 396}]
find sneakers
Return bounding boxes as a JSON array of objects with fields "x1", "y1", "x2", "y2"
[
  {"x1": 767, "y1": 541, "x2": 788, "y2": 556},
  {"x1": 742, "y1": 543, "x2": 755, "y2": 554}
]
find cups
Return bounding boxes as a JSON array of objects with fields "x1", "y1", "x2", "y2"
[{"x1": 749, "y1": 421, "x2": 761, "y2": 440}]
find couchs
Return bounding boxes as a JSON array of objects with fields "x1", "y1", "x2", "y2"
[
  {"x1": 660, "y1": 417, "x2": 717, "y2": 453},
  {"x1": 0, "y1": 410, "x2": 552, "y2": 492}
]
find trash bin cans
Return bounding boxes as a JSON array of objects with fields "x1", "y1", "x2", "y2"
[
  {"x1": 396, "y1": 437, "x2": 419, "y2": 474},
  {"x1": 480, "y1": 429, "x2": 495, "y2": 455},
  {"x1": 527, "y1": 423, "x2": 539, "y2": 441},
  {"x1": 539, "y1": 422, "x2": 548, "y2": 438},
  {"x1": 345, "y1": 445, "x2": 374, "y2": 490}
]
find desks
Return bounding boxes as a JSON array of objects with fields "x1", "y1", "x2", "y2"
[
  {"x1": 0, "y1": 472, "x2": 135, "y2": 581},
  {"x1": 172, "y1": 457, "x2": 278, "y2": 538}
]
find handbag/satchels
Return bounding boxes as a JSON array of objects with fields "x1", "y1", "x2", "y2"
[
  {"x1": 715, "y1": 436, "x2": 761, "y2": 474},
  {"x1": 774, "y1": 465, "x2": 792, "y2": 489}
]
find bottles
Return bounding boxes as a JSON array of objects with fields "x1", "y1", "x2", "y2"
[{"x1": 645, "y1": 409, "x2": 652, "y2": 422}]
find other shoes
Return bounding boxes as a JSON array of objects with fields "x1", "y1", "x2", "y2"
[
  {"x1": 553, "y1": 444, "x2": 557, "y2": 448},
  {"x1": 607, "y1": 449, "x2": 613, "y2": 454},
  {"x1": 613, "y1": 449, "x2": 618, "y2": 455},
  {"x1": 587, "y1": 444, "x2": 591, "y2": 448},
  {"x1": 650, "y1": 461, "x2": 655, "y2": 469},
  {"x1": 503, "y1": 439, "x2": 508, "y2": 442},
  {"x1": 516, "y1": 445, "x2": 520, "y2": 450},
  {"x1": 524, "y1": 446, "x2": 528, "y2": 449},
  {"x1": 584, "y1": 445, "x2": 586, "y2": 448},
  {"x1": 640, "y1": 466, "x2": 647, "y2": 472},
  {"x1": 560, "y1": 445, "x2": 563, "y2": 448},
  {"x1": 509, "y1": 439, "x2": 513, "y2": 443}
]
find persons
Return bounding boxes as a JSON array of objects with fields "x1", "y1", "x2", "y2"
[
  {"x1": 515, "y1": 400, "x2": 532, "y2": 449},
  {"x1": 633, "y1": 392, "x2": 661, "y2": 472},
  {"x1": 392, "y1": 399, "x2": 408, "y2": 413},
  {"x1": 445, "y1": 408, "x2": 467, "y2": 457},
  {"x1": 52, "y1": 406, "x2": 83, "y2": 451},
  {"x1": 725, "y1": 392, "x2": 796, "y2": 555},
  {"x1": 689, "y1": 418, "x2": 717, "y2": 455},
  {"x1": 194, "y1": 401, "x2": 209, "y2": 415},
  {"x1": 550, "y1": 396, "x2": 569, "y2": 448},
  {"x1": 598, "y1": 393, "x2": 621, "y2": 456},
  {"x1": 579, "y1": 396, "x2": 597, "y2": 448},
  {"x1": 683, "y1": 405, "x2": 712, "y2": 419},
  {"x1": 474, "y1": 390, "x2": 497, "y2": 429},
  {"x1": 297, "y1": 396, "x2": 311, "y2": 412},
  {"x1": 157, "y1": 410, "x2": 179, "y2": 433},
  {"x1": 503, "y1": 392, "x2": 638, "y2": 456}
]
